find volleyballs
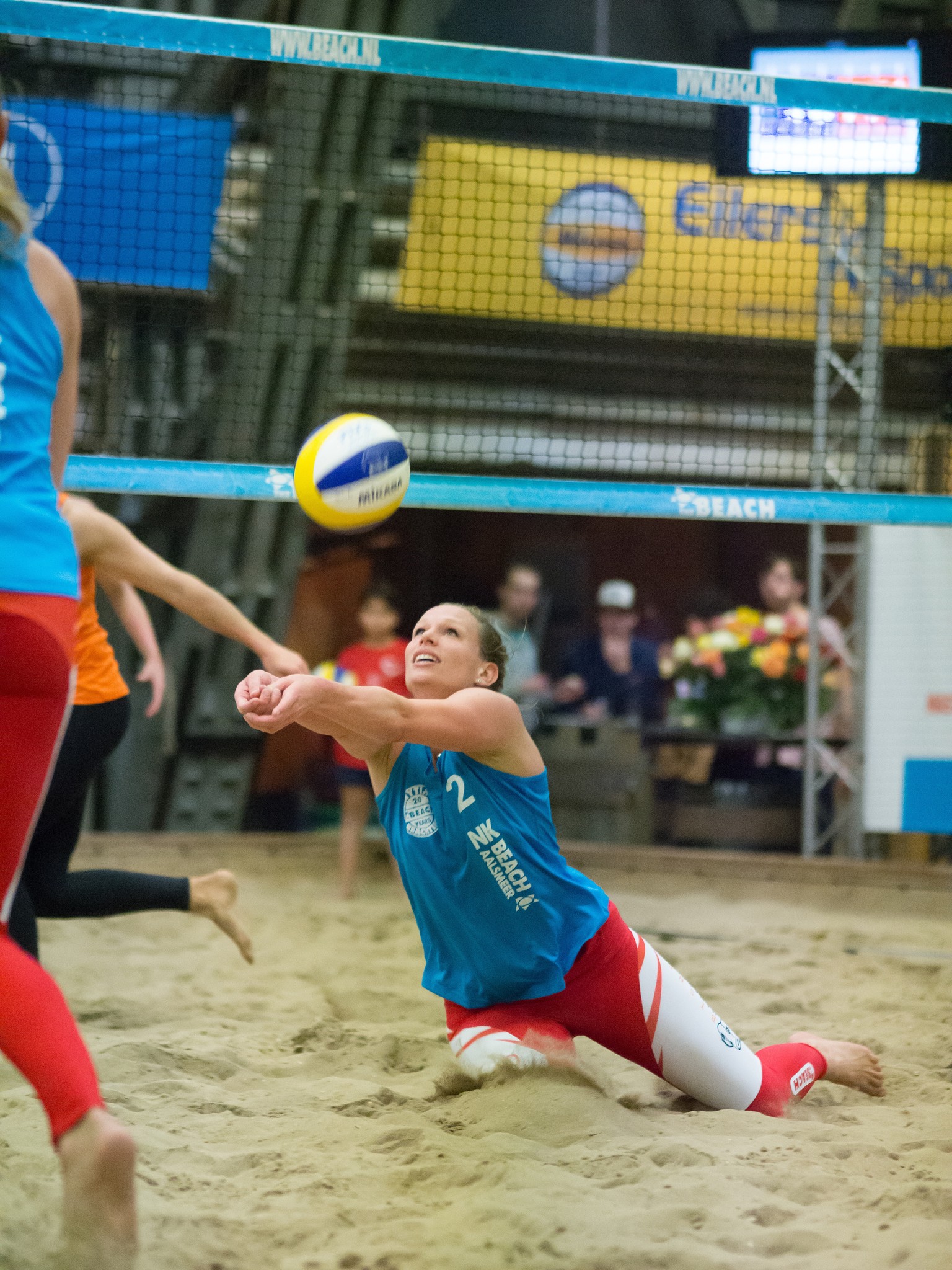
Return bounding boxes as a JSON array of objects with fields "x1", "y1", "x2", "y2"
[{"x1": 288, "y1": 413, "x2": 411, "y2": 532}]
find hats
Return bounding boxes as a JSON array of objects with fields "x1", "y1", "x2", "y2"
[{"x1": 595, "y1": 577, "x2": 637, "y2": 610}]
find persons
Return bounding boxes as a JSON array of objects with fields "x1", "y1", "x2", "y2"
[
  {"x1": 236, "y1": 600, "x2": 886, "y2": 1117},
  {"x1": 327, "y1": 551, "x2": 852, "y2": 902},
  {"x1": 0, "y1": 105, "x2": 143, "y2": 1270},
  {"x1": 6, "y1": 493, "x2": 318, "y2": 971}
]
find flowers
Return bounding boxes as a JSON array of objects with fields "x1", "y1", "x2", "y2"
[{"x1": 656, "y1": 604, "x2": 848, "y2": 737}]
